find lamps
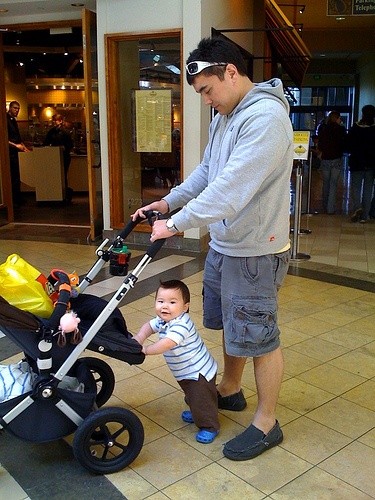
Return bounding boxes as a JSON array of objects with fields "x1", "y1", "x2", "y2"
[
  {"x1": 278, "y1": 5, "x2": 305, "y2": 14},
  {"x1": 293, "y1": 23, "x2": 303, "y2": 31}
]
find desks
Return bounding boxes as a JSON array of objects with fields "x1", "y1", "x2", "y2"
[{"x1": 33, "y1": 146, "x2": 66, "y2": 207}]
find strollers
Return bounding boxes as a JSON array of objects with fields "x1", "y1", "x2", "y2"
[{"x1": 0, "y1": 208, "x2": 169, "y2": 476}]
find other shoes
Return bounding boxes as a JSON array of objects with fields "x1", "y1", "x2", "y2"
[
  {"x1": 222, "y1": 419, "x2": 283, "y2": 460},
  {"x1": 328, "y1": 213, "x2": 335, "y2": 215},
  {"x1": 360, "y1": 216, "x2": 370, "y2": 222},
  {"x1": 183, "y1": 384, "x2": 247, "y2": 411},
  {"x1": 350, "y1": 207, "x2": 363, "y2": 222}
]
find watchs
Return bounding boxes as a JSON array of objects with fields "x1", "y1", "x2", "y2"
[{"x1": 164, "y1": 218, "x2": 179, "y2": 234}]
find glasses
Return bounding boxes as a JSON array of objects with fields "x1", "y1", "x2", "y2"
[{"x1": 185, "y1": 61, "x2": 230, "y2": 76}]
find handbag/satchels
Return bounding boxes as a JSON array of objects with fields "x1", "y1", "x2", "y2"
[{"x1": 0, "y1": 253, "x2": 71, "y2": 319}]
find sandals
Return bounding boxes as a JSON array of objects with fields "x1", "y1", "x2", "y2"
[
  {"x1": 182, "y1": 410, "x2": 194, "y2": 422},
  {"x1": 195, "y1": 429, "x2": 219, "y2": 443}
]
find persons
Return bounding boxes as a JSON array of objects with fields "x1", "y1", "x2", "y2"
[
  {"x1": 132, "y1": 278, "x2": 222, "y2": 444},
  {"x1": 311, "y1": 111, "x2": 348, "y2": 215},
  {"x1": 7, "y1": 101, "x2": 32, "y2": 216},
  {"x1": 39, "y1": 113, "x2": 74, "y2": 187},
  {"x1": 343, "y1": 105, "x2": 375, "y2": 223},
  {"x1": 131, "y1": 37, "x2": 294, "y2": 461}
]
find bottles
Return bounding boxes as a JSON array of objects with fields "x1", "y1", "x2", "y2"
[{"x1": 109, "y1": 241, "x2": 130, "y2": 276}]
